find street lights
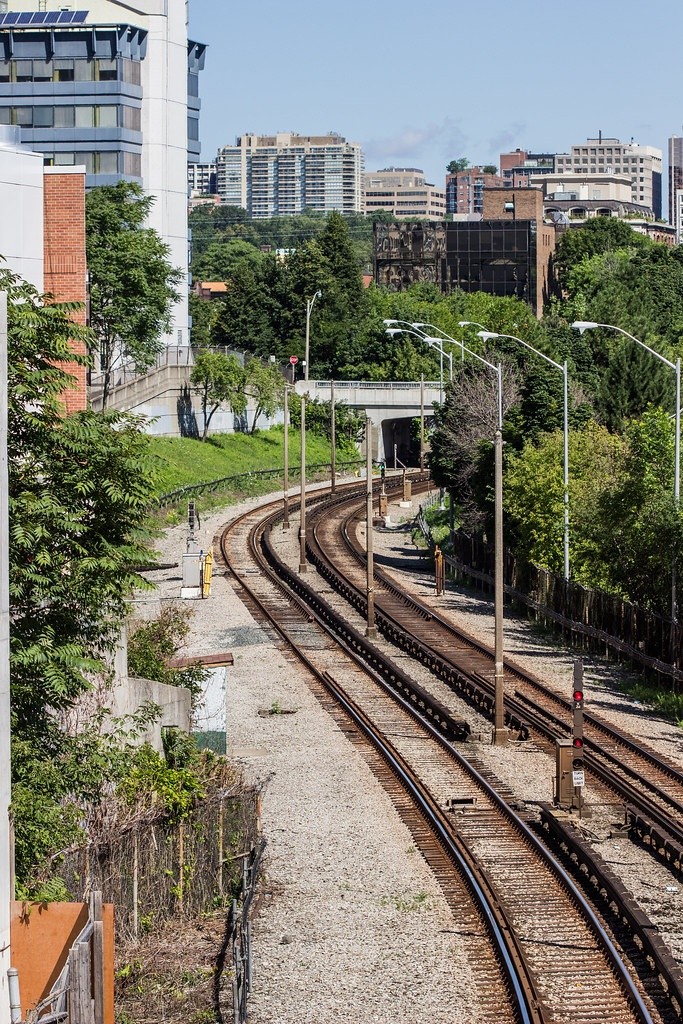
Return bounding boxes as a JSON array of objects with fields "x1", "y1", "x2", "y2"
[
  {"x1": 382, "y1": 320, "x2": 455, "y2": 412},
  {"x1": 572, "y1": 322, "x2": 680, "y2": 655},
  {"x1": 474, "y1": 330, "x2": 573, "y2": 583},
  {"x1": 304, "y1": 290, "x2": 322, "y2": 382},
  {"x1": 421, "y1": 337, "x2": 512, "y2": 748}
]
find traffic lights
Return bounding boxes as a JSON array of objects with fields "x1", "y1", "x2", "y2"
[{"x1": 381, "y1": 465, "x2": 386, "y2": 480}]
